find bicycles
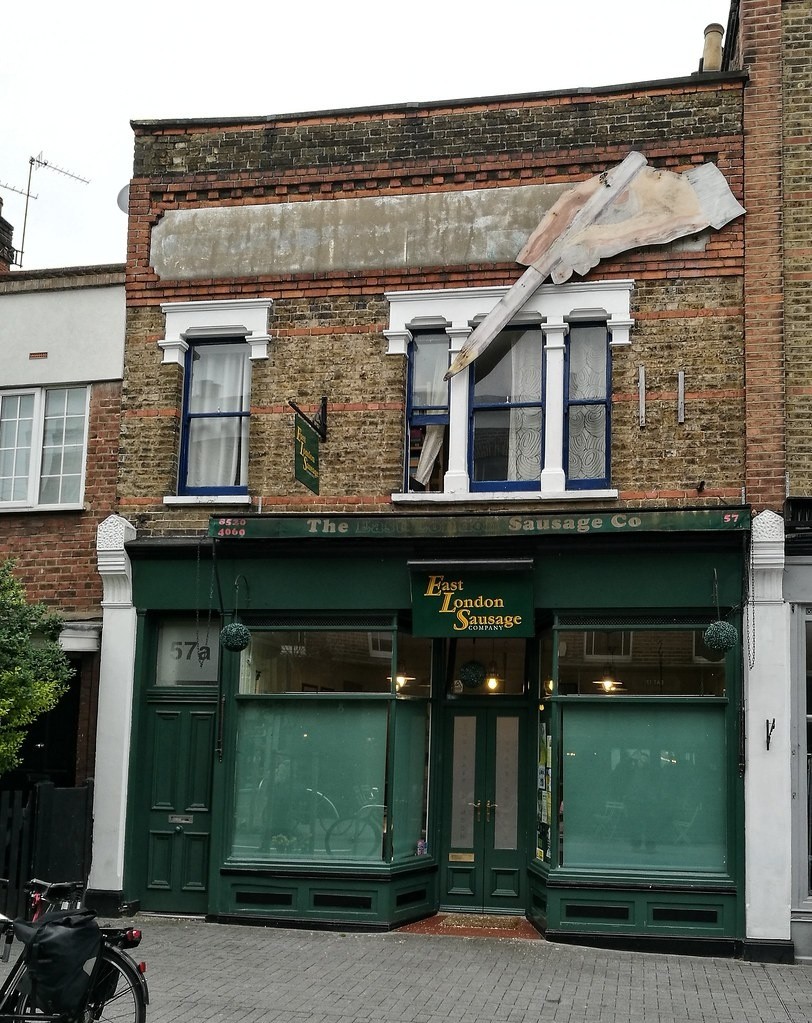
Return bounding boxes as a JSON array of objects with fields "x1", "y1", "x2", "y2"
[{"x1": 0, "y1": 873, "x2": 149, "y2": 1023}]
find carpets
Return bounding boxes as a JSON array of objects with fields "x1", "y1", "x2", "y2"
[{"x1": 440, "y1": 915, "x2": 523, "y2": 930}]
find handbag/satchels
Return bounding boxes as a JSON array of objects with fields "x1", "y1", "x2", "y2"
[{"x1": 23, "y1": 909, "x2": 102, "y2": 1016}]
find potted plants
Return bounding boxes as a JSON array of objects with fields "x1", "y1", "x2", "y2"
[{"x1": 219, "y1": 622, "x2": 251, "y2": 652}]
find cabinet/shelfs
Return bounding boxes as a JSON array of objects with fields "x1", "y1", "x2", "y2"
[{"x1": 410, "y1": 427, "x2": 443, "y2": 491}]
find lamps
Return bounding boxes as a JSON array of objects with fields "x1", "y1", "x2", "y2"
[
  {"x1": 593, "y1": 630, "x2": 628, "y2": 693},
  {"x1": 482, "y1": 639, "x2": 506, "y2": 689},
  {"x1": 387, "y1": 634, "x2": 417, "y2": 689}
]
